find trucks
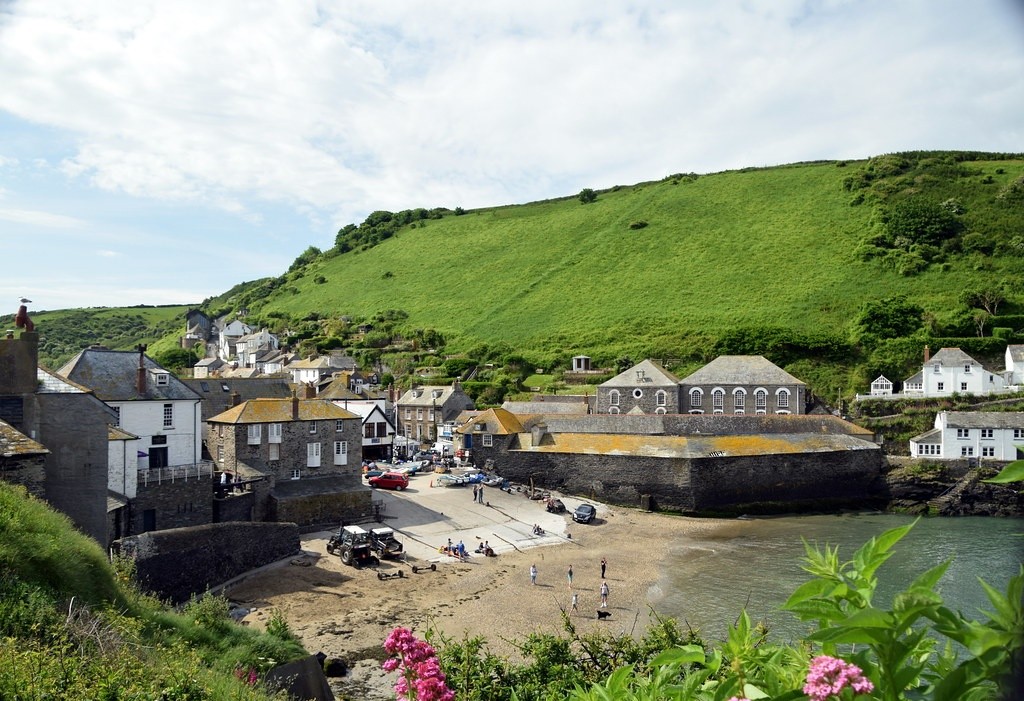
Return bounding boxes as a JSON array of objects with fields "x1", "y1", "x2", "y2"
[{"x1": 370, "y1": 526, "x2": 402, "y2": 560}]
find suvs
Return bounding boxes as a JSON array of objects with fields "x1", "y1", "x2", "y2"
[{"x1": 367, "y1": 472, "x2": 409, "y2": 492}]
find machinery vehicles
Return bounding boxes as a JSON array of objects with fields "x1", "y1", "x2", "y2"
[{"x1": 327, "y1": 526, "x2": 381, "y2": 570}]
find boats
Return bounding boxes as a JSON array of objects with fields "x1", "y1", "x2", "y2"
[{"x1": 375, "y1": 461, "x2": 504, "y2": 487}]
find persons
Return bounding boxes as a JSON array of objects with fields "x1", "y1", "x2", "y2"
[
  {"x1": 567, "y1": 564, "x2": 573, "y2": 589},
  {"x1": 449, "y1": 484, "x2": 558, "y2": 562},
  {"x1": 601, "y1": 557, "x2": 608, "y2": 578},
  {"x1": 567, "y1": 594, "x2": 578, "y2": 616},
  {"x1": 599, "y1": 582, "x2": 610, "y2": 608},
  {"x1": 530, "y1": 564, "x2": 538, "y2": 586}
]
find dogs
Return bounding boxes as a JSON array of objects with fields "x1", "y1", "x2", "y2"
[{"x1": 597, "y1": 611, "x2": 612, "y2": 620}]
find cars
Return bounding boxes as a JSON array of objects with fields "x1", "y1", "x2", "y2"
[{"x1": 573, "y1": 503, "x2": 596, "y2": 524}]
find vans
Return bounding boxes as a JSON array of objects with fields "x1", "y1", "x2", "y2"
[{"x1": 429, "y1": 441, "x2": 453, "y2": 458}]
